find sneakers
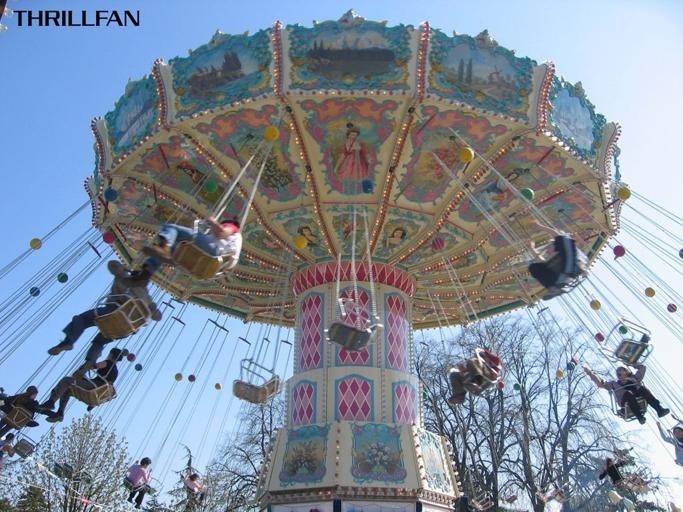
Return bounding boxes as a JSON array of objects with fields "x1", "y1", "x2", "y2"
[
  {"x1": 121, "y1": 275, "x2": 149, "y2": 289},
  {"x1": 45, "y1": 411, "x2": 63, "y2": 423},
  {"x1": 35, "y1": 399, "x2": 55, "y2": 411},
  {"x1": 639, "y1": 407, "x2": 670, "y2": 425},
  {"x1": 127, "y1": 496, "x2": 141, "y2": 509},
  {"x1": 448, "y1": 381, "x2": 483, "y2": 404},
  {"x1": 542, "y1": 273, "x2": 571, "y2": 301},
  {"x1": 73, "y1": 360, "x2": 94, "y2": 378},
  {"x1": 141, "y1": 243, "x2": 174, "y2": 266},
  {"x1": 48, "y1": 340, "x2": 73, "y2": 355}
]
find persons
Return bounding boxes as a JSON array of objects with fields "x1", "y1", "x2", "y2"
[
  {"x1": 448, "y1": 347, "x2": 500, "y2": 405},
  {"x1": 298, "y1": 224, "x2": 320, "y2": 249},
  {"x1": 582, "y1": 361, "x2": 670, "y2": 425},
  {"x1": 332, "y1": 120, "x2": 374, "y2": 184},
  {"x1": 599, "y1": 458, "x2": 638, "y2": 507},
  {"x1": 383, "y1": 225, "x2": 406, "y2": 250},
  {"x1": 656, "y1": 413, "x2": 683, "y2": 468},
  {"x1": 470, "y1": 169, "x2": 520, "y2": 211},
  {"x1": 126, "y1": 456, "x2": 153, "y2": 510},
  {"x1": 183, "y1": 165, "x2": 224, "y2": 204},
  {"x1": 526, "y1": 219, "x2": 588, "y2": 301},
  {"x1": 0, "y1": 432, "x2": 16, "y2": 470},
  {"x1": 184, "y1": 471, "x2": 209, "y2": 506},
  {"x1": 248, "y1": 133, "x2": 290, "y2": 193}
]
